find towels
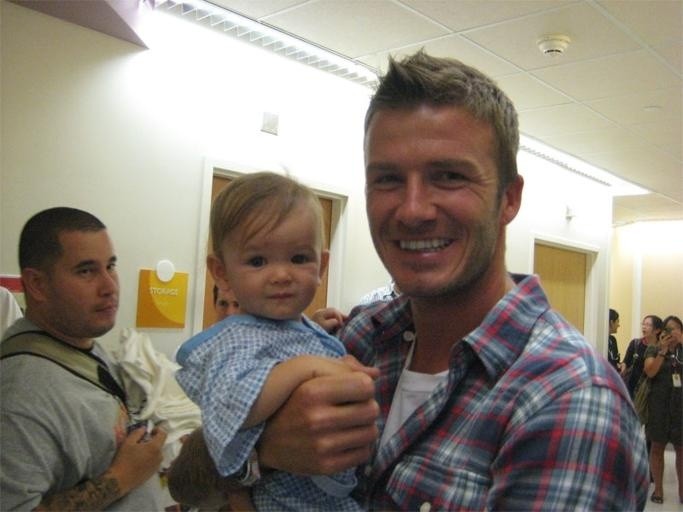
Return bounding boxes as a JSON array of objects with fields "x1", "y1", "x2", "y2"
[{"x1": 118, "y1": 328, "x2": 202, "y2": 446}]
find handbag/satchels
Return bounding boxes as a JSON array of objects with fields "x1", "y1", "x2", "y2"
[{"x1": 632, "y1": 377, "x2": 649, "y2": 424}]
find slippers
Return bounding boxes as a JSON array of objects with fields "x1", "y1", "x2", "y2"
[{"x1": 651, "y1": 491, "x2": 663, "y2": 503}]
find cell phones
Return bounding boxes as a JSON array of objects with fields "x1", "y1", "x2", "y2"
[{"x1": 664, "y1": 328, "x2": 672, "y2": 339}]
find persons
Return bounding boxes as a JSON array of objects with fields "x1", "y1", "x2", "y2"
[
  {"x1": 0, "y1": 206, "x2": 167, "y2": 512},
  {"x1": 255, "y1": 47, "x2": 651, "y2": 512},
  {"x1": 608, "y1": 308, "x2": 683, "y2": 504},
  {"x1": 211, "y1": 283, "x2": 240, "y2": 325},
  {"x1": 167, "y1": 173, "x2": 357, "y2": 511}
]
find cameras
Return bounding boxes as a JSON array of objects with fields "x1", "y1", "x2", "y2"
[{"x1": 128, "y1": 419, "x2": 154, "y2": 442}]
